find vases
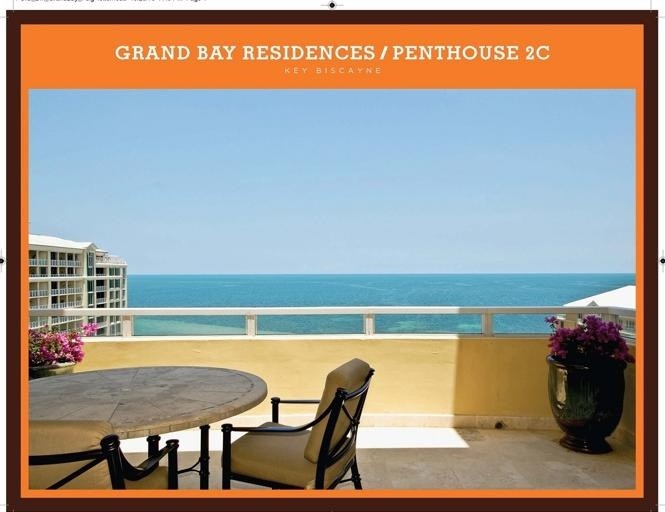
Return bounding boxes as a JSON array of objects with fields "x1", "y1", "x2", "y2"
[
  {"x1": 545, "y1": 355, "x2": 625, "y2": 455},
  {"x1": 29, "y1": 362, "x2": 77, "y2": 379}
]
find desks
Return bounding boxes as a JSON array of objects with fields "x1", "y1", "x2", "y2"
[{"x1": 29, "y1": 366, "x2": 268, "y2": 490}]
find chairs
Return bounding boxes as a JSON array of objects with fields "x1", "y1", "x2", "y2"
[
  {"x1": 220, "y1": 357, "x2": 375, "y2": 491},
  {"x1": 30, "y1": 417, "x2": 181, "y2": 491}
]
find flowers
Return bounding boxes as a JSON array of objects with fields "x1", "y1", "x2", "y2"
[
  {"x1": 29, "y1": 322, "x2": 98, "y2": 365},
  {"x1": 543, "y1": 312, "x2": 636, "y2": 368}
]
